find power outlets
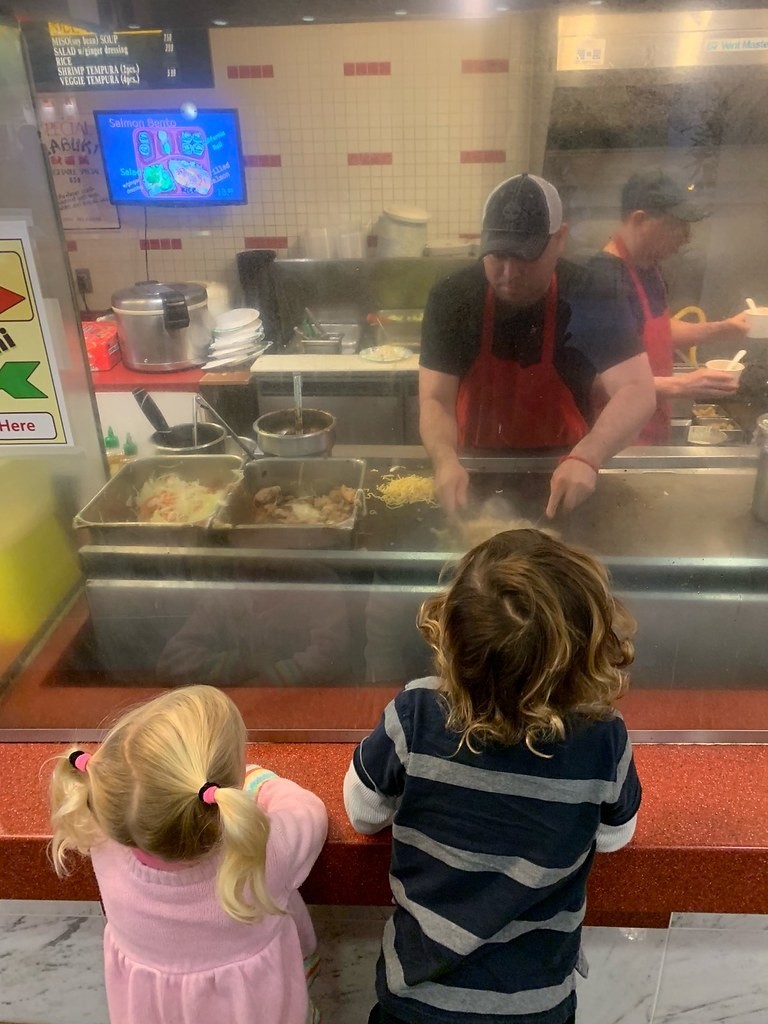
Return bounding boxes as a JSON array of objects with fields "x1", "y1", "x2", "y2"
[{"x1": 75, "y1": 268, "x2": 93, "y2": 294}]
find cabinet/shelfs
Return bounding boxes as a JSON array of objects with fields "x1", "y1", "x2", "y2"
[{"x1": 249, "y1": 339, "x2": 697, "y2": 445}]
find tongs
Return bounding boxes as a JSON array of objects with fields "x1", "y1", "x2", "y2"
[{"x1": 192, "y1": 394, "x2": 255, "y2": 459}]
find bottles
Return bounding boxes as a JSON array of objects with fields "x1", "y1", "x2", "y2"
[
  {"x1": 120, "y1": 432, "x2": 141, "y2": 470},
  {"x1": 377, "y1": 207, "x2": 429, "y2": 257},
  {"x1": 104, "y1": 427, "x2": 122, "y2": 478}
]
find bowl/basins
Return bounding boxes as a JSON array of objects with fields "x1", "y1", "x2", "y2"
[
  {"x1": 149, "y1": 421, "x2": 227, "y2": 454},
  {"x1": 744, "y1": 306, "x2": 768, "y2": 338},
  {"x1": 253, "y1": 409, "x2": 336, "y2": 456},
  {"x1": 705, "y1": 359, "x2": 745, "y2": 384},
  {"x1": 225, "y1": 435, "x2": 256, "y2": 458}
]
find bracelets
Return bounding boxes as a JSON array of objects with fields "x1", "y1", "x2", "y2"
[{"x1": 560, "y1": 455, "x2": 598, "y2": 472}]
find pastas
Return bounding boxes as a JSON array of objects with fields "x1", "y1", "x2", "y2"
[{"x1": 364, "y1": 473, "x2": 439, "y2": 510}]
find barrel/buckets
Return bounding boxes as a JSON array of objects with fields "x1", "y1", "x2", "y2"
[{"x1": 752, "y1": 412, "x2": 768, "y2": 524}]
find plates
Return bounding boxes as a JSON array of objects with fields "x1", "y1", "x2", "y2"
[
  {"x1": 201, "y1": 308, "x2": 273, "y2": 371},
  {"x1": 360, "y1": 345, "x2": 413, "y2": 362},
  {"x1": 132, "y1": 126, "x2": 213, "y2": 196}
]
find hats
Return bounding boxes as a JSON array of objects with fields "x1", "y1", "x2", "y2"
[
  {"x1": 620, "y1": 167, "x2": 715, "y2": 223},
  {"x1": 478, "y1": 173, "x2": 564, "y2": 261}
]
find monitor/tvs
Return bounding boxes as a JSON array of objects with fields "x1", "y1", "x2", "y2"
[{"x1": 93, "y1": 108, "x2": 248, "y2": 207}]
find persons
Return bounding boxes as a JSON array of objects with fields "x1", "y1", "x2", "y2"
[
  {"x1": 340, "y1": 529, "x2": 644, "y2": 1024},
  {"x1": 587, "y1": 168, "x2": 754, "y2": 446},
  {"x1": 49, "y1": 684, "x2": 329, "y2": 1024},
  {"x1": 419, "y1": 174, "x2": 658, "y2": 525}
]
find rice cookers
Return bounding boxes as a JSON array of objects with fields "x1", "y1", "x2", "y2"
[{"x1": 111, "y1": 280, "x2": 213, "y2": 373}]
